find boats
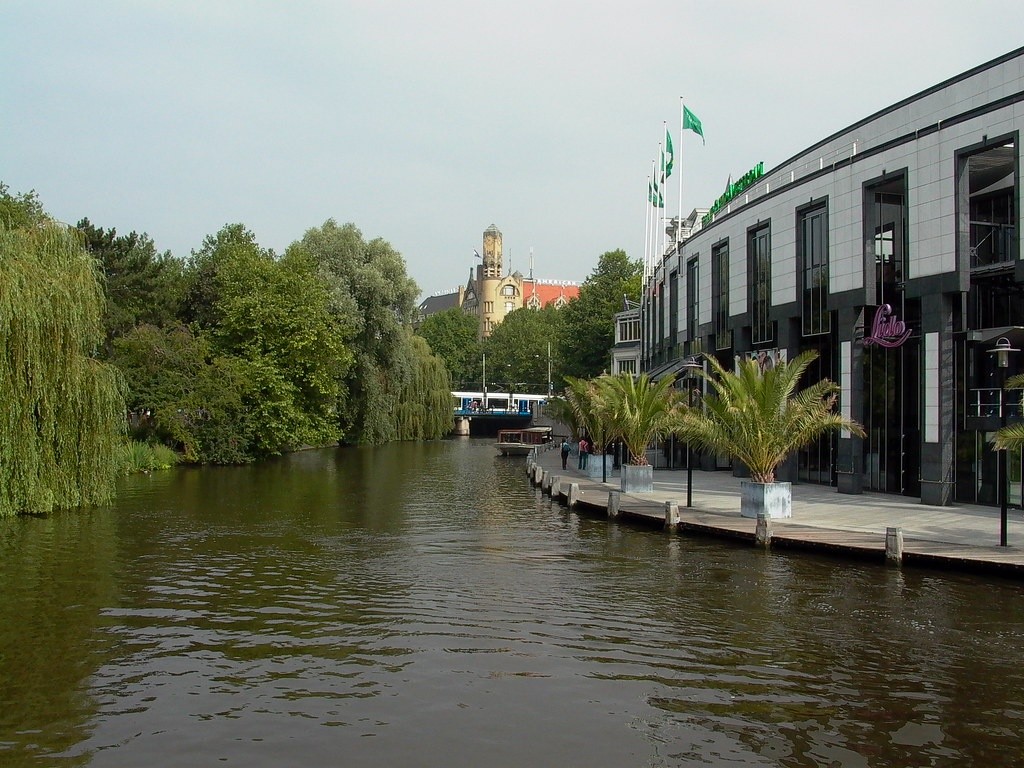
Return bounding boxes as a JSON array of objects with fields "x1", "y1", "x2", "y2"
[{"x1": 493, "y1": 426, "x2": 555, "y2": 455}]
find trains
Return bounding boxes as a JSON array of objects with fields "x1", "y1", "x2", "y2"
[{"x1": 449, "y1": 391, "x2": 566, "y2": 415}]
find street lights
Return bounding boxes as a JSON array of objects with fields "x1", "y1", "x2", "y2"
[
  {"x1": 986, "y1": 337, "x2": 1021, "y2": 547},
  {"x1": 681, "y1": 356, "x2": 705, "y2": 507}
]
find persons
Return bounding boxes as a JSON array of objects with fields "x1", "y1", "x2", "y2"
[
  {"x1": 578, "y1": 437, "x2": 588, "y2": 469},
  {"x1": 745, "y1": 351, "x2": 773, "y2": 372},
  {"x1": 515, "y1": 403, "x2": 517, "y2": 410},
  {"x1": 558, "y1": 438, "x2": 569, "y2": 470},
  {"x1": 474, "y1": 402, "x2": 495, "y2": 413}
]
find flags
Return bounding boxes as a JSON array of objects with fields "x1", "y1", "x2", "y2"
[{"x1": 649, "y1": 104, "x2": 704, "y2": 208}]
